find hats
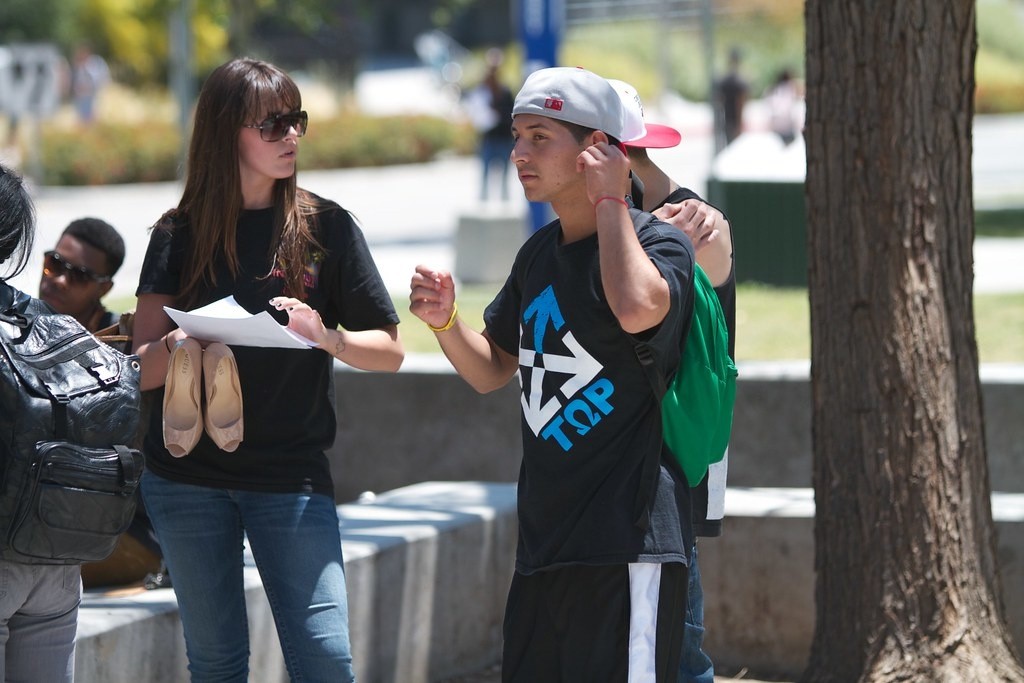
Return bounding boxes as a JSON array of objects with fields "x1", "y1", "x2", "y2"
[
  {"x1": 512, "y1": 67, "x2": 628, "y2": 158},
  {"x1": 605, "y1": 79, "x2": 682, "y2": 149}
]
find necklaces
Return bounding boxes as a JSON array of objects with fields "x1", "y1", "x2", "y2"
[{"x1": 237, "y1": 238, "x2": 277, "y2": 281}]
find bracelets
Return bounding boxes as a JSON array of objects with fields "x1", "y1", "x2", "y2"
[
  {"x1": 427, "y1": 304, "x2": 458, "y2": 332},
  {"x1": 594, "y1": 196, "x2": 629, "y2": 208},
  {"x1": 165, "y1": 335, "x2": 171, "y2": 353}
]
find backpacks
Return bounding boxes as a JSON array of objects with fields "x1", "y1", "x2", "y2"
[
  {"x1": 512, "y1": 170, "x2": 738, "y2": 487},
  {"x1": 0, "y1": 291, "x2": 144, "y2": 565}
]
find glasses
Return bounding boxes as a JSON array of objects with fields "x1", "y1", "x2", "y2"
[
  {"x1": 241, "y1": 111, "x2": 308, "y2": 142},
  {"x1": 44, "y1": 250, "x2": 112, "y2": 289}
]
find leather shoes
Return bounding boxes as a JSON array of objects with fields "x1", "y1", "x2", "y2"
[
  {"x1": 162, "y1": 337, "x2": 201, "y2": 458},
  {"x1": 202, "y1": 343, "x2": 245, "y2": 454}
]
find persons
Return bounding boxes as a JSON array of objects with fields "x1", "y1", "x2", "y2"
[
  {"x1": 0, "y1": 165, "x2": 171, "y2": 683},
  {"x1": 718, "y1": 47, "x2": 749, "y2": 141},
  {"x1": 471, "y1": 58, "x2": 516, "y2": 200},
  {"x1": 409, "y1": 67, "x2": 737, "y2": 683},
  {"x1": 132, "y1": 57, "x2": 403, "y2": 683}
]
